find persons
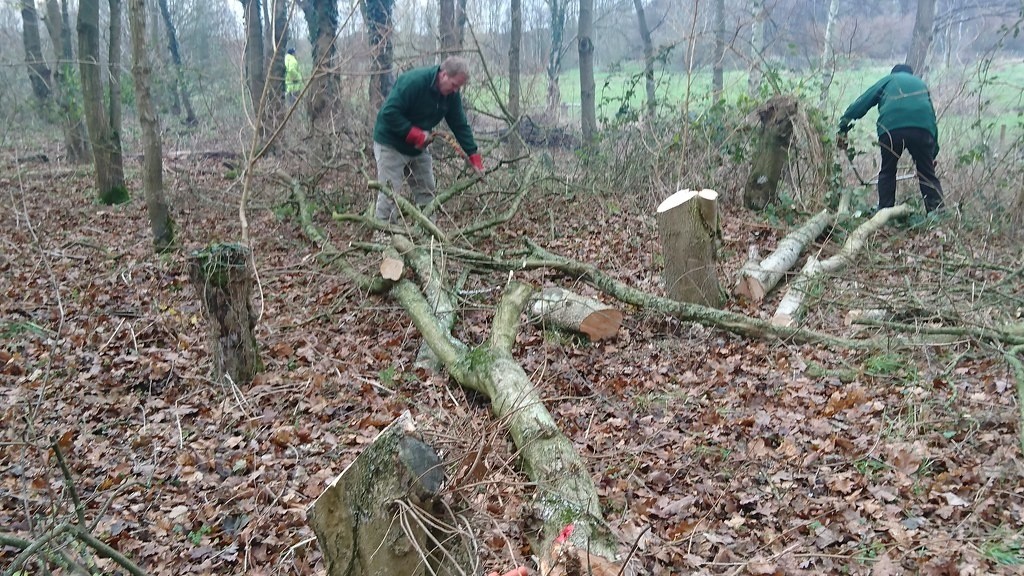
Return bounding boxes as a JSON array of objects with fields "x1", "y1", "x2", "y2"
[
  {"x1": 836, "y1": 64, "x2": 946, "y2": 216},
  {"x1": 285, "y1": 48, "x2": 304, "y2": 106},
  {"x1": 372, "y1": 57, "x2": 486, "y2": 224}
]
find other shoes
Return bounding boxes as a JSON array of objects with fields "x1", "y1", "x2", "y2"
[{"x1": 371, "y1": 229, "x2": 391, "y2": 244}]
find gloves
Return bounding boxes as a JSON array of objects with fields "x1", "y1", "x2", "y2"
[
  {"x1": 468, "y1": 154, "x2": 485, "y2": 180},
  {"x1": 406, "y1": 126, "x2": 435, "y2": 151},
  {"x1": 838, "y1": 121, "x2": 848, "y2": 137}
]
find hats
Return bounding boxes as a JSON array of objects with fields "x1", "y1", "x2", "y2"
[{"x1": 891, "y1": 65, "x2": 913, "y2": 74}]
